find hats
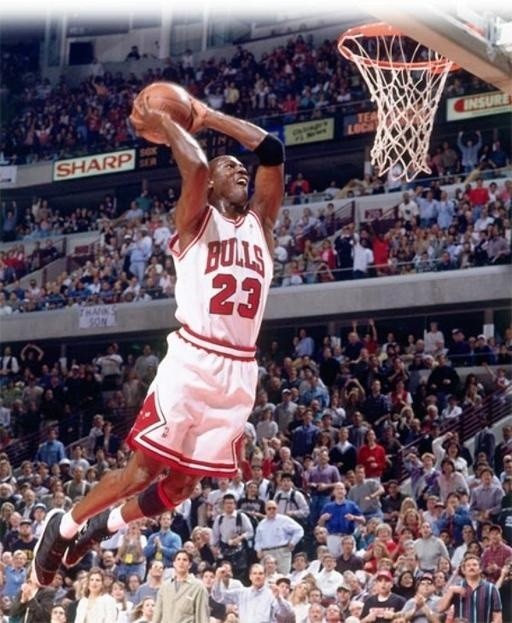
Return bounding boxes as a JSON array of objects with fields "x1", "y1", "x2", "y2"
[{"x1": 377, "y1": 570, "x2": 393, "y2": 581}]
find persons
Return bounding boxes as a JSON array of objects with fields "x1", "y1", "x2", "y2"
[
  {"x1": 0, "y1": 322, "x2": 512, "y2": 622},
  {"x1": 0, "y1": 130, "x2": 512, "y2": 315},
  {"x1": 33, "y1": 94, "x2": 284, "y2": 587},
  {"x1": 2, "y1": 19, "x2": 499, "y2": 167}
]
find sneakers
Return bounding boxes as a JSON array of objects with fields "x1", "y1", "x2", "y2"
[{"x1": 31, "y1": 507, "x2": 118, "y2": 588}]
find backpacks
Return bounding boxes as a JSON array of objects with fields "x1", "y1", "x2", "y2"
[
  {"x1": 219, "y1": 510, "x2": 259, "y2": 541},
  {"x1": 275, "y1": 488, "x2": 312, "y2": 510}
]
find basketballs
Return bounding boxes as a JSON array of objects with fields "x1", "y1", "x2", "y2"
[{"x1": 133, "y1": 81, "x2": 193, "y2": 146}]
